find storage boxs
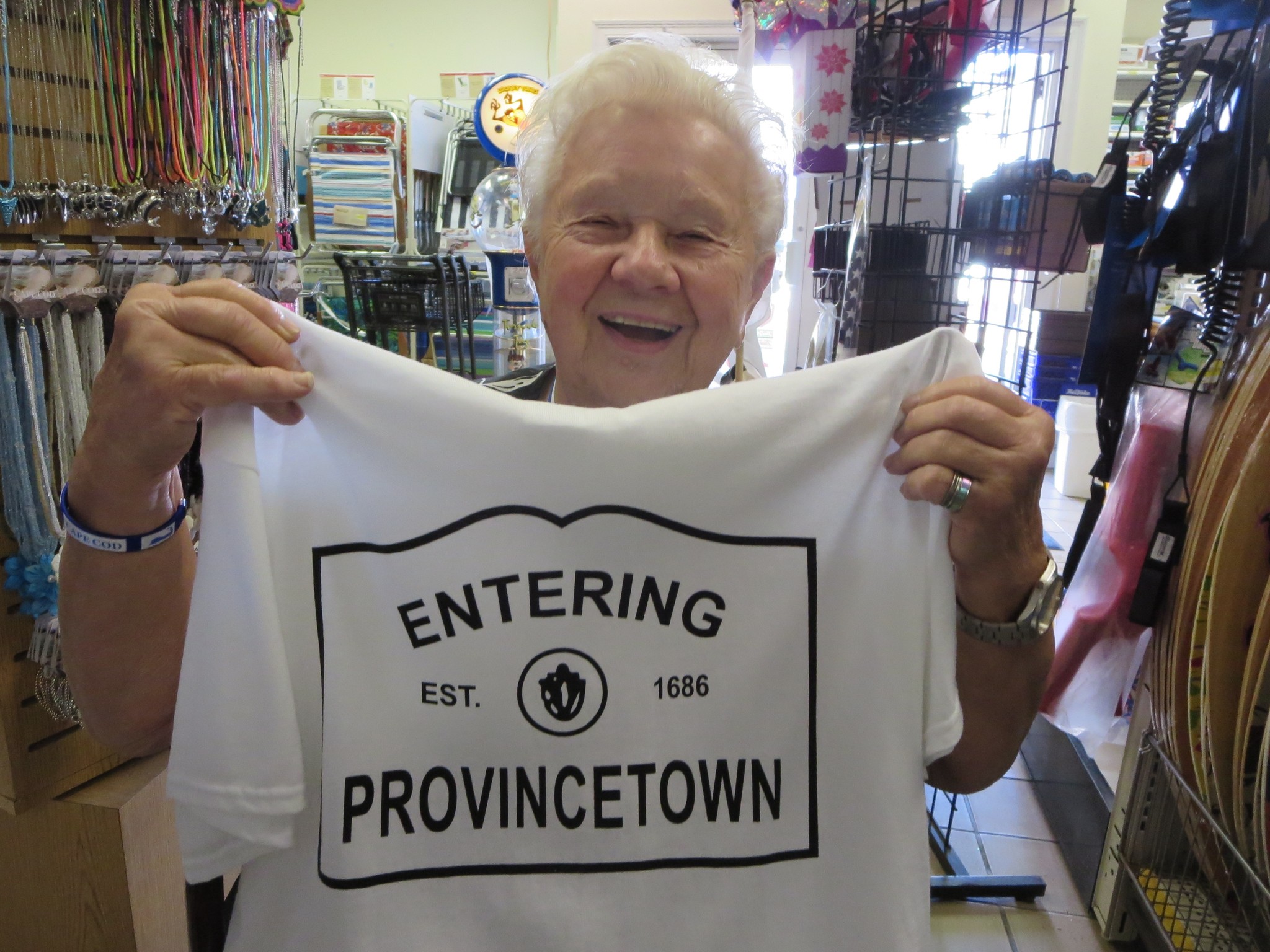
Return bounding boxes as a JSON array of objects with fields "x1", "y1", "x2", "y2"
[
  {"x1": 1108, "y1": 45, "x2": 1156, "y2": 166},
  {"x1": 814, "y1": 230, "x2": 938, "y2": 356},
  {"x1": 1011, "y1": 309, "x2": 1103, "y2": 500},
  {"x1": 0, "y1": 532, "x2": 137, "y2": 815},
  {"x1": 969, "y1": 180, "x2": 1098, "y2": 273}
]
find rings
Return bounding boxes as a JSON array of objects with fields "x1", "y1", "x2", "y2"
[{"x1": 942, "y1": 471, "x2": 973, "y2": 512}]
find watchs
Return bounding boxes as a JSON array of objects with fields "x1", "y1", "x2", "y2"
[{"x1": 956, "y1": 548, "x2": 1064, "y2": 646}]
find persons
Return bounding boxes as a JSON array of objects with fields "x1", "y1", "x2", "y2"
[{"x1": 53, "y1": 38, "x2": 1057, "y2": 795}]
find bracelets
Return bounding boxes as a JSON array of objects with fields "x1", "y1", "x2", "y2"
[{"x1": 58, "y1": 481, "x2": 187, "y2": 553}]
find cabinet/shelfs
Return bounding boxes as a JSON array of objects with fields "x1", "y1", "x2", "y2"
[
  {"x1": 1039, "y1": 0, "x2": 1270, "y2": 713},
  {"x1": 809, "y1": 0, "x2": 1075, "y2": 905},
  {"x1": 1108, "y1": 71, "x2": 1208, "y2": 173}
]
